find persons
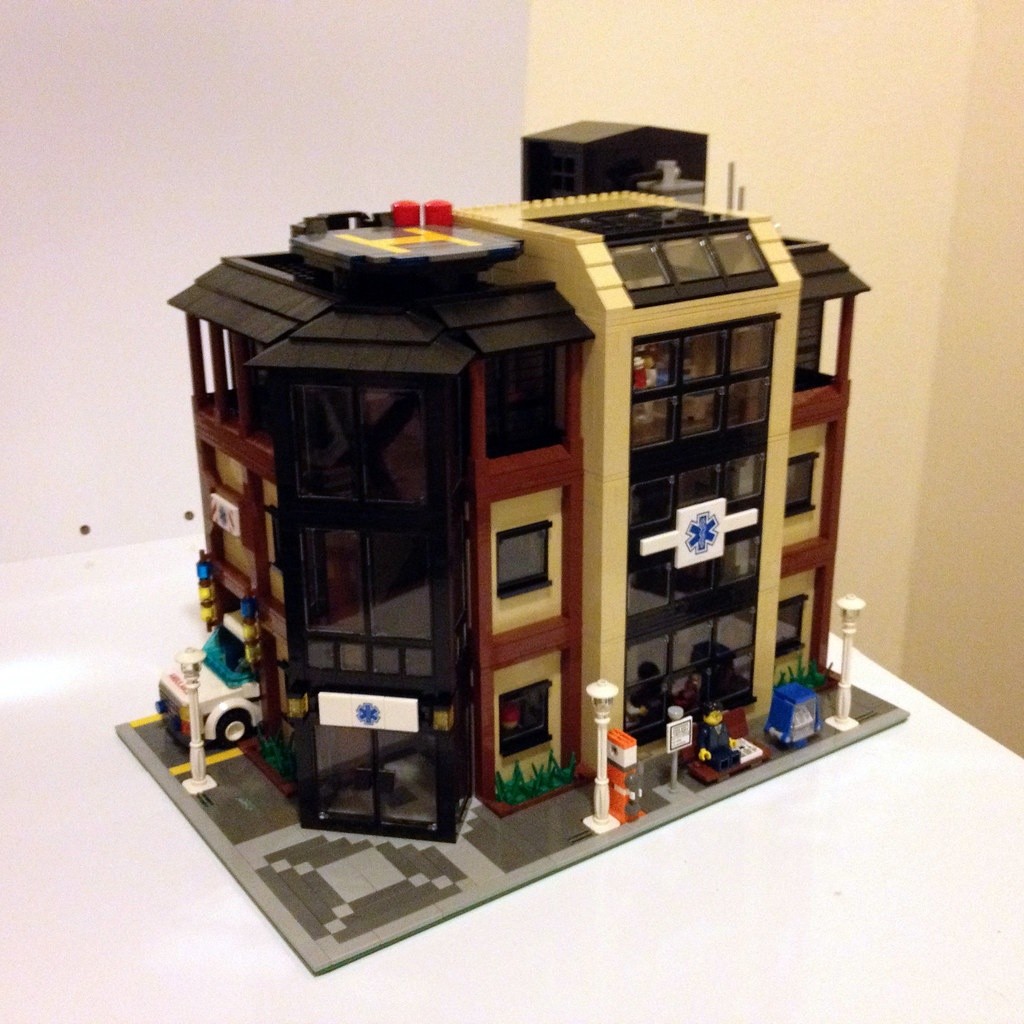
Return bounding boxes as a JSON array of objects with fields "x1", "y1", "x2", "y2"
[{"x1": 698, "y1": 700, "x2": 741, "y2": 772}]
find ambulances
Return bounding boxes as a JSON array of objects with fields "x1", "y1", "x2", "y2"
[{"x1": 154, "y1": 612, "x2": 264, "y2": 755}]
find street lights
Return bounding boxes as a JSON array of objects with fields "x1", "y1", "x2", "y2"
[
  {"x1": 823, "y1": 593, "x2": 861, "y2": 732},
  {"x1": 582, "y1": 679, "x2": 621, "y2": 835},
  {"x1": 175, "y1": 646, "x2": 217, "y2": 795}
]
